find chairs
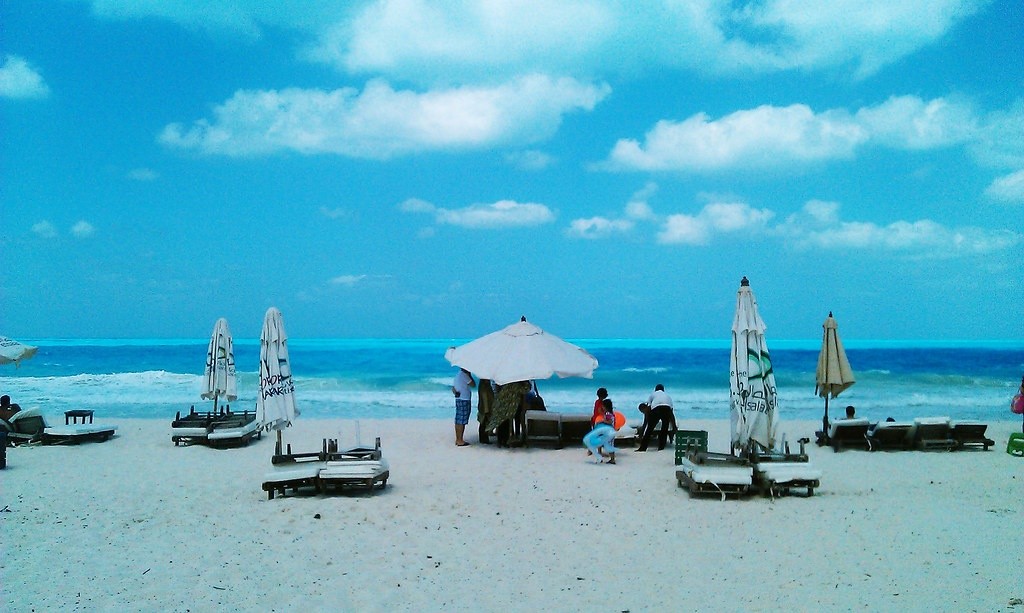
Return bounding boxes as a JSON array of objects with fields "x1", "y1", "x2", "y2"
[
  {"x1": 868, "y1": 421, "x2": 912, "y2": 449},
  {"x1": 170, "y1": 402, "x2": 262, "y2": 447},
  {"x1": 262, "y1": 429, "x2": 390, "y2": 502},
  {"x1": 675, "y1": 437, "x2": 823, "y2": 499},
  {"x1": 525, "y1": 410, "x2": 592, "y2": 450},
  {"x1": 815, "y1": 418, "x2": 872, "y2": 453},
  {"x1": 42, "y1": 423, "x2": 118, "y2": 446},
  {"x1": 950, "y1": 419, "x2": 995, "y2": 451},
  {"x1": 8, "y1": 405, "x2": 50, "y2": 447},
  {"x1": 914, "y1": 416, "x2": 954, "y2": 449},
  {"x1": 615, "y1": 420, "x2": 674, "y2": 449}
]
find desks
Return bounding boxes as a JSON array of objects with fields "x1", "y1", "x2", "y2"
[{"x1": 63, "y1": 409, "x2": 95, "y2": 425}]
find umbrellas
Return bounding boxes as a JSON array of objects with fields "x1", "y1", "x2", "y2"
[
  {"x1": 815, "y1": 311, "x2": 855, "y2": 447},
  {"x1": 730, "y1": 277, "x2": 779, "y2": 484},
  {"x1": 200, "y1": 318, "x2": 237, "y2": 411},
  {"x1": 0, "y1": 336, "x2": 38, "y2": 364},
  {"x1": 257, "y1": 307, "x2": 298, "y2": 455},
  {"x1": 445, "y1": 316, "x2": 599, "y2": 385}
]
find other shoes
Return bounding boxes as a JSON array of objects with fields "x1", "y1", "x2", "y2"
[
  {"x1": 634, "y1": 448, "x2": 646, "y2": 452},
  {"x1": 605, "y1": 459, "x2": 615, "y2": 464}
]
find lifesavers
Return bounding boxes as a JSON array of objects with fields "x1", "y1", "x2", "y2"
[
  {"x1": 594, "y1": 410, "x2": 626, "y2": 431},
  {"x1": 583, "y1": 423, "x2": 619, "y2": 464}
]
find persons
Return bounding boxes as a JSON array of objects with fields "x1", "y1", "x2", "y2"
[
  {"x1": 845, "y1": 406, "x2": 855, "y2": 418},
  {"x1": 588, "y1": 388, "x2": 615, "y2": 464},
  {"x1": 0, "y1": 395, "x2": 21, "y2": 420},
  {"x1": 477, "y1": 379, "x2": 540, "y2": 448},
  {"x1": 452, "y1": 369, "x2": 476, "y2": 446},
  {"x1": 634, "y1": 384, "x2": 677, "y2": 453}
]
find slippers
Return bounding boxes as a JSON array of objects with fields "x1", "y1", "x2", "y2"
[{"x1": 458, "y1": 442, "x2": 470, "y2": 446}]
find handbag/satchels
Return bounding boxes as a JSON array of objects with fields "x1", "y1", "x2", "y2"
[{"x1": 1010, "y1": 386, "x2": 1024, "y2": 414}]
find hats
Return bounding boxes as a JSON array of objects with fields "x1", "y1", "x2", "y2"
[{"x1": 638, "y1": 402, "x2": 648, "y2": 411}]
